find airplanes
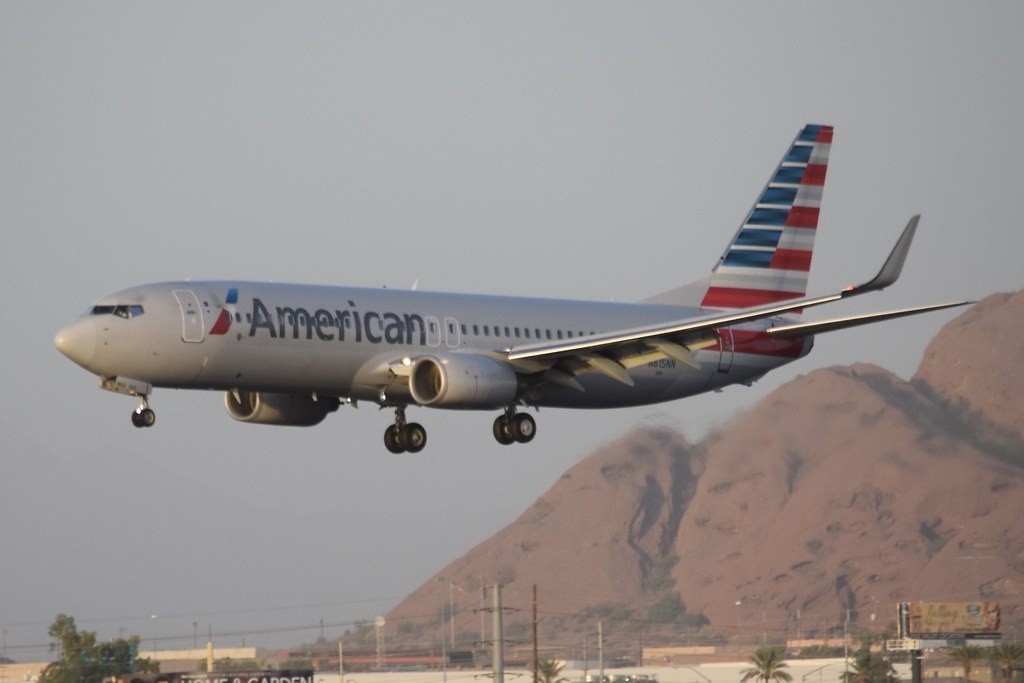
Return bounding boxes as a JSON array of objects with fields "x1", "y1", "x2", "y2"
[{"x1": 53, "y1": 123, "x2": 976, "y2": 455}]
